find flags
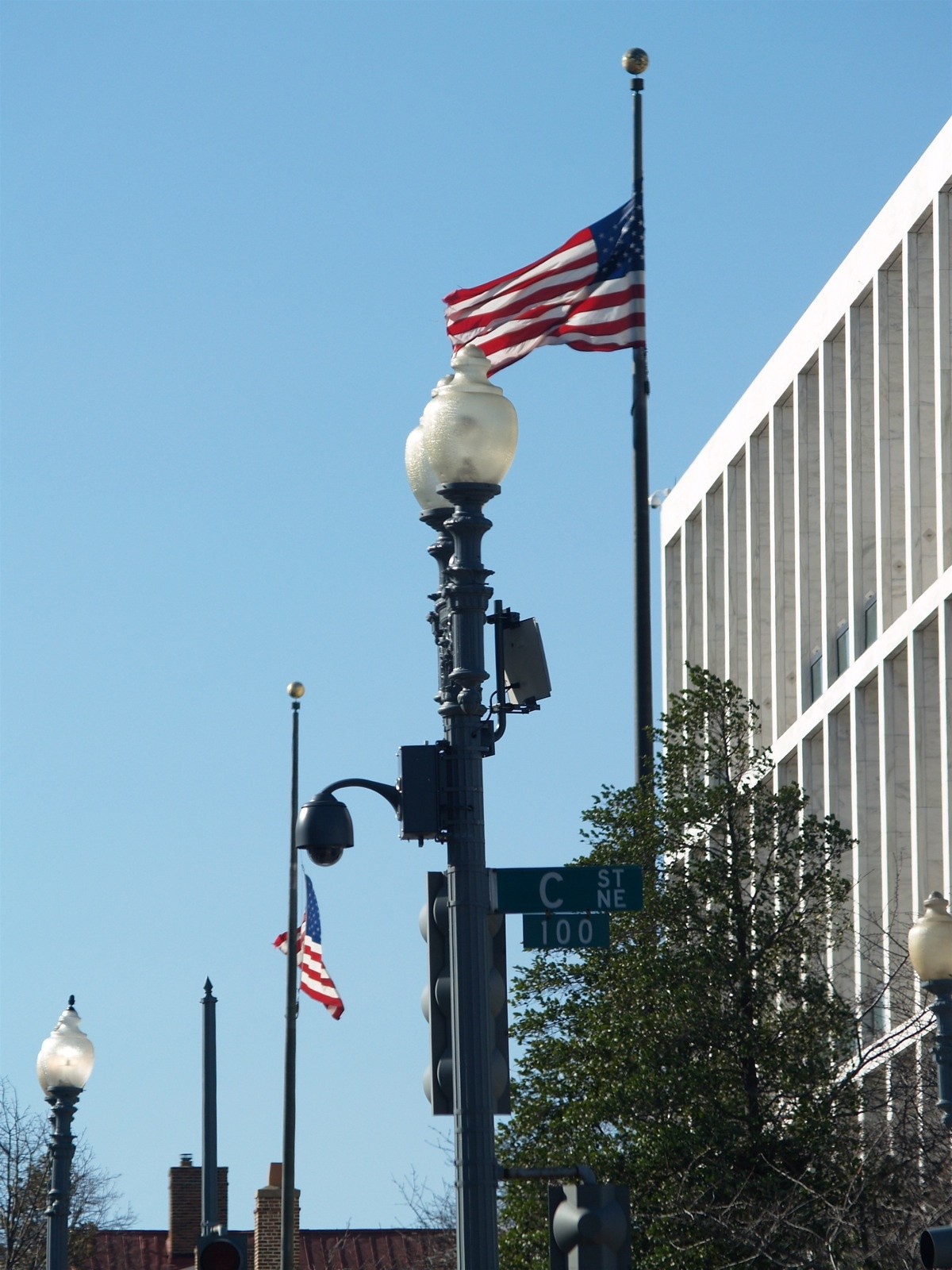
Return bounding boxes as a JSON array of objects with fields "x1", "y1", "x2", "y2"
[
  {"x1": 271, "y1": 872, "x2": 345, "y2": 1022},
  {"x1": 442, "y1": 189, "x2": 645, "y2": 378}
]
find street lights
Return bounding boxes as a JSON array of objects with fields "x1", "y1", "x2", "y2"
[
  {"x1": 905, "y1": 888, "x2": 952, "y2": 1127},
  {"x1": 293, "y1": 736, "x2": 512, "y2": 1269},
  {"x1": 35, "y1": 993, "x2": 96, "y2": 1270},
  {"x1": 294, "y1": 341, "x2": 521, "y2": 1269}
]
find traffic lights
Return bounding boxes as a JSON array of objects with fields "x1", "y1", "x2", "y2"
[{"x1": 917, "y1": 1222, "x2": 952, "y2": 1270}]
